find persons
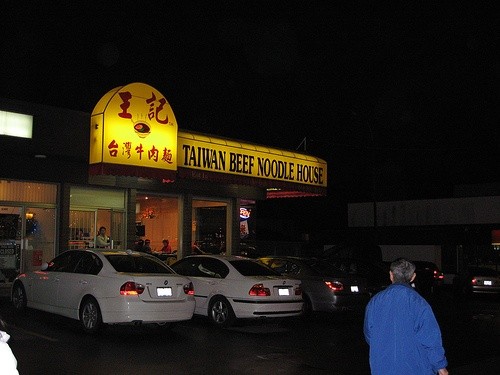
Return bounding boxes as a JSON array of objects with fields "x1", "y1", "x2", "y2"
[
  {"x1": 363, "y1": 258, "x2": 449, "y2": 375},
  {"x1": 97, "y1": 227, "x2": 110, "y2": 249},
  {"x1": 134, "y1": 239, "x2": 152, "y2": 254},
  {"x1": 0, "y1": 300, "x2": 24, "y2": 375},
  {"x1": 76, "y1": 230, "x2": 85, "y2": 240},
  {"x1": 160, "y1": 239, "x2": 171, "y2": 254}
]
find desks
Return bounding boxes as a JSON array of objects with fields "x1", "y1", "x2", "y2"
[{"x1": 153, "y1": 253, "x2": 175, "y2": 259}]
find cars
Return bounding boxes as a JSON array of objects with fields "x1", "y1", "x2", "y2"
[
  {"x1": 167, "y1": 254, "x2": 305, "y2": 329},
  {"x1": 11, "y1": 249, "x2": 196, "y2": 338},
  {"x1": 452, "y1": 265, "x2": 500, "y2": 295},
  {"x1": 255, "y1": 254, "x2": 369, "y2": 319},
  {"x1": 410, "y1": 260, "x2": 445, "y2": 293}
]
